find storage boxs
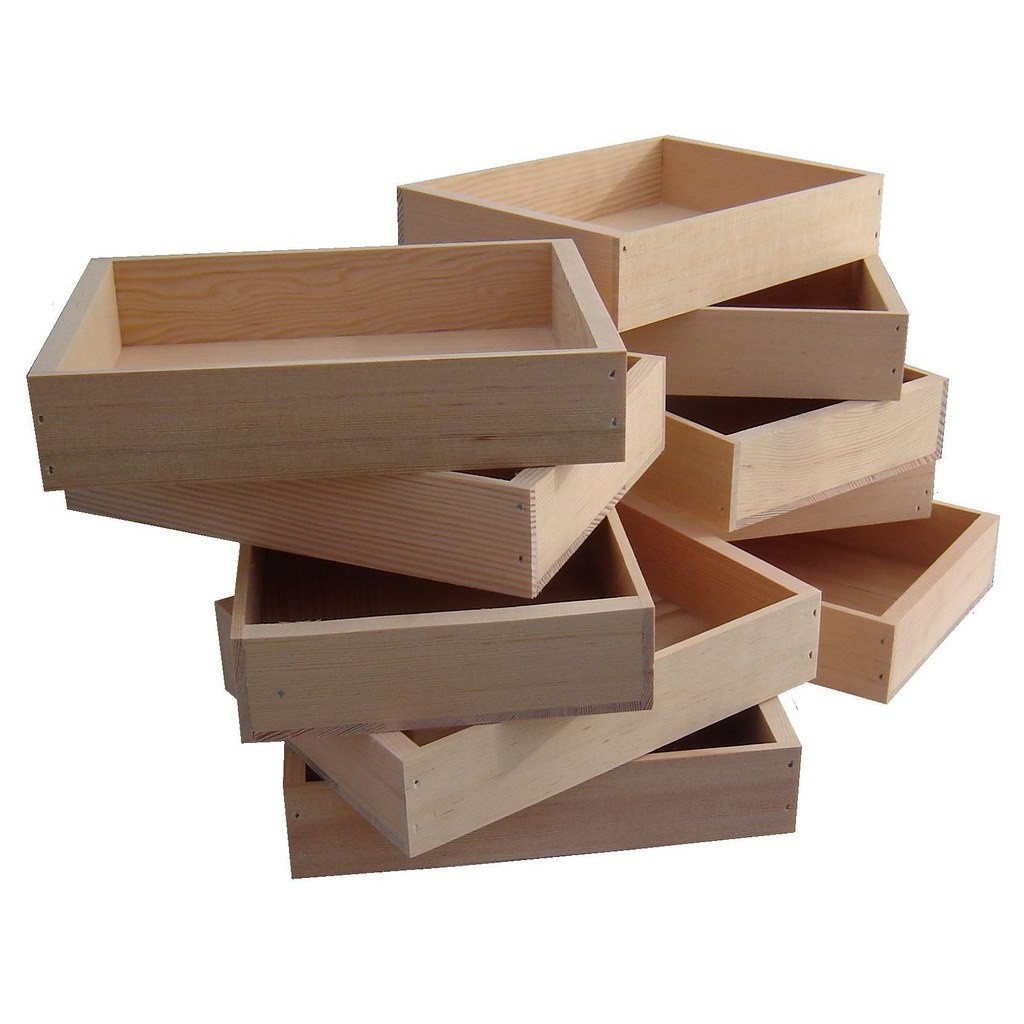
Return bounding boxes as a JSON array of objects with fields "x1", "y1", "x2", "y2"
[
  {"x1": 617, "y1": 254, "x2": 911, "y2": 403},
  {"x1": 394, "y1": 133, "x2": 886, "y2": 337},
  {"x1": 726, "y1": 500, "x2": 1001, "y2": 705},
  {"x1": 213, "y1": 492, "x2": 823, "y2": 863},
  {"x1": 280, "y1": 695, "x2": 804, "y2": 881},
  {"x1": 22, "y1": 233, "x2": 628, "y2": 492},
  {"x1": 61, "y1": 348, "x2": 669, "y2": 601},
  {"x1": 228, "y1": 508, "x2": 659, "y2": 744},
  {"x1": 633, "y1": 363, "x2": 952, "y2": 543}
]
